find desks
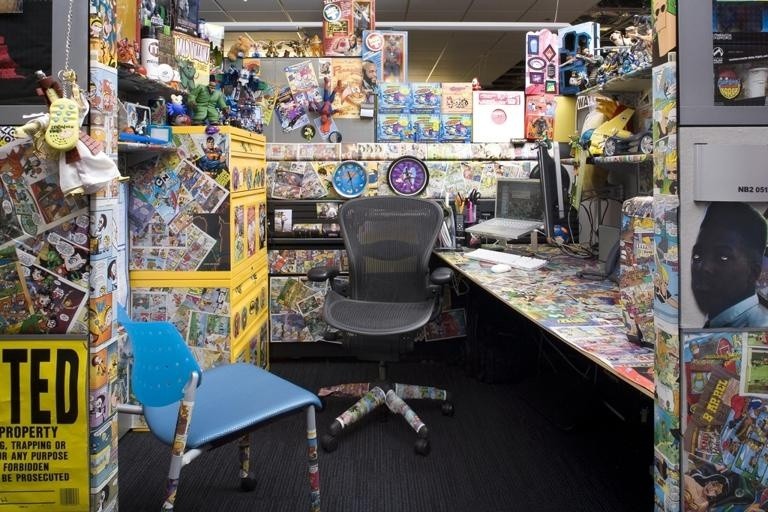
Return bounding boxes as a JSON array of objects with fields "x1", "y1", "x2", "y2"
[{"x1": 433, "y1": 244, "x2": 656, "y2": 399}]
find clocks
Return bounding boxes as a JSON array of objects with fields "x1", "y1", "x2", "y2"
[
  {"x1": 387, "y1": 155, "x2": 430, "y2": 195},
  {"x1": 331, "y1": 159, "x2": 368, "y2": 198}
]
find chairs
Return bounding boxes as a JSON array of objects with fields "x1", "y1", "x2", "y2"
[
  {"x1": 307, "y1": 195, "x2": 455, "y2": 456},
  {"x1": 118, "y1": 302, "x2": 324, "y2": 512}
]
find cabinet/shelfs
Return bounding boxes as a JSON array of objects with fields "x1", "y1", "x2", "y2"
[
  {"x1": 560, "y1": 61, "x2": 653, "y2": 174},
  {"x1": 267, "y1": 234, "x2": 349, "y2": 361},
  {"x1": 126, "y1": 125, "x2": 270, "y2": 433}
]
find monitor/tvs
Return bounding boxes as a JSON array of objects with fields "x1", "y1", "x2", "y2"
[{"x1": 537, "y1": 147, "x2": 570, "y2": 238}]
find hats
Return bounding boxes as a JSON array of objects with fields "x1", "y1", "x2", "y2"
[{"x1": 701, "y1": 201, "x2": 768, "y2": 255}]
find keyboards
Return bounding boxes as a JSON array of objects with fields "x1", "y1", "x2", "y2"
[{"x1": 463, "y1": 248, "x2": 548, "y2": 270}]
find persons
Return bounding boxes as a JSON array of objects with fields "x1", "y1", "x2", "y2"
[
  {"x1": 116, "y1": 0, "x2": 653, "y2": 157},
  {"x1": 688, "y1": 201, "x2": 768, "y2": 329},
  {"x1": 682, "y1": 472, "x2": 729, "y2": 511},
  {"x1": 15, "y1": 68, "x2": 131, "y2": 198},
  {"x1": 199, "y1": 137, "x2": 228, "y2": 171}
]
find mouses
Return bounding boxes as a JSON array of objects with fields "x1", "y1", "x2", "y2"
[{"x1": 491, "y1": 263, "x2": 512, "y2": 274}]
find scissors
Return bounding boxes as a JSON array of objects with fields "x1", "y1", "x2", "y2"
[{"x1": 470, "y1": 189, "x2": 480, "y2": 200}]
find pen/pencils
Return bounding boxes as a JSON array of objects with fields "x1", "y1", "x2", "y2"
[
  {"x1": 568, "y1": 294, "x2": 578, "y2": 302},
  {"x1": 455, "y1": 193, "x2": 466, "y2": 214}
]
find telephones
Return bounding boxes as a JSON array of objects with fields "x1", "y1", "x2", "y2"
[{"x1": 603, "y1": 239, "x2": 620, "y2": 283}]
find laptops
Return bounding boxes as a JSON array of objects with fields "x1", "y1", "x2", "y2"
[{"x1": 464, "y1": 177, "x2": 545, "y2": 240}]
